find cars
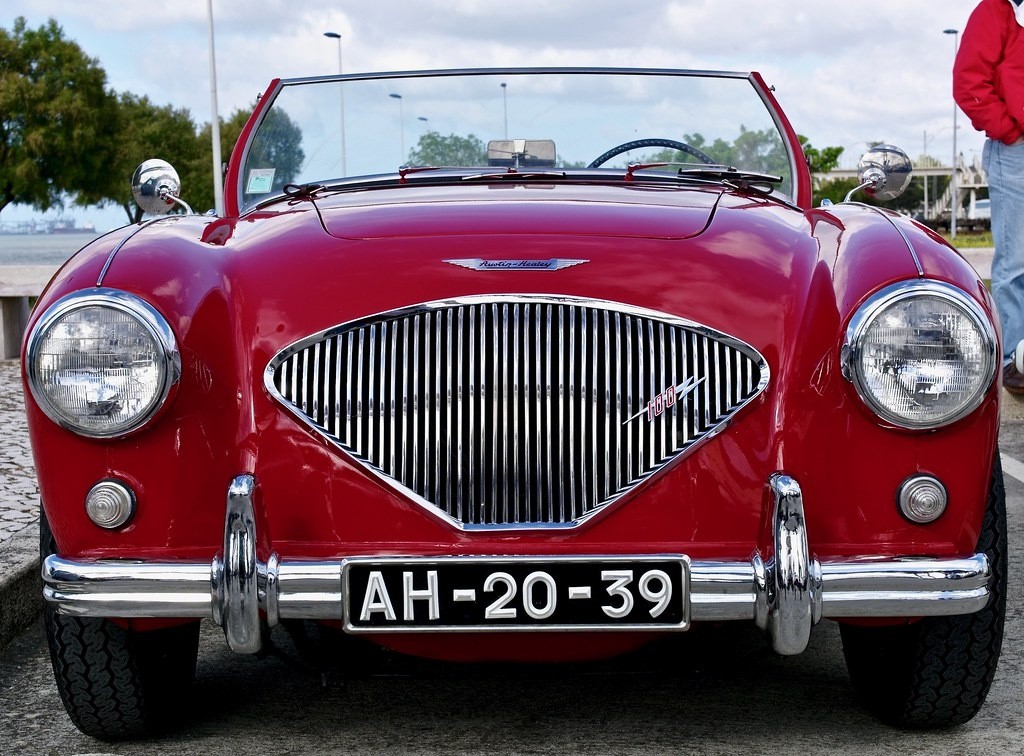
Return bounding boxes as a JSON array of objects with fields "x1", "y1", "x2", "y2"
[
  {"x1": 965, "y1": 200, "x2": 992, "y2": 230},
  {"x1": 18, "y1": 67, "x2": 1011, "y2": 741}
]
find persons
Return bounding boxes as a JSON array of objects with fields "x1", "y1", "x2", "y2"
[{"x1": 953, "y1": 0, "x2": 1024, "y2": 395}]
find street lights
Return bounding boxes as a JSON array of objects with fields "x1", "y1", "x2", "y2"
[
  {"x1": 323, "y1": 31, "x2": 347, "y2": 178},
  {"x1": 418, "y1": 117, "x2": 429, "y2": 137},
  {"x1": 389, "y1": 92, "x2": 406, "y2": 161},
  {"x1": 945, "y1": 27, "x2": 958, "y2": 241},
  {"x1": 501, "y1": 82, "x2": 508, "y2": 141}
]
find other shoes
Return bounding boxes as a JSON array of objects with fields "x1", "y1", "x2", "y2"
[{"x1": 1002, "y1": 362, "x2": 1024, "y2": 396}]
